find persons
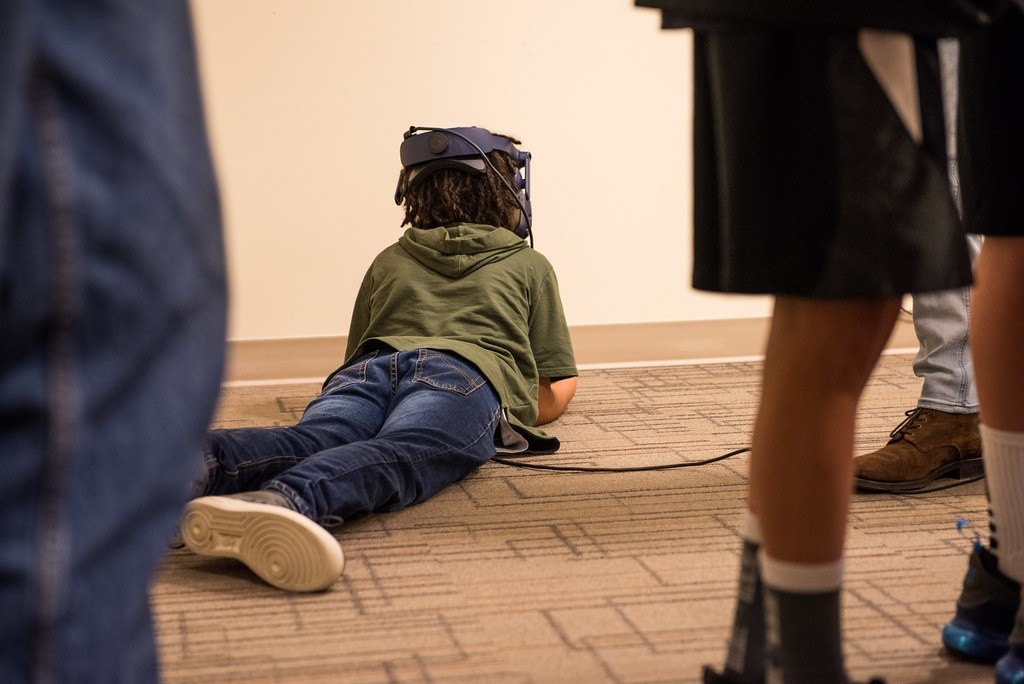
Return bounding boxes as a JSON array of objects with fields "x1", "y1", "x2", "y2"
[
  {"x1": 623, "y1": 0, "x2": 1024, "y2": 684},
  {"x1": 168, "y1": 126, "x2": 579, "y2": 593},
  {"x1": 0, "y1": 0, "x2": 232, "y2": 684}
]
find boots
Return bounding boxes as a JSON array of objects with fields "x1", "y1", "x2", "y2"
[{"x1": 764, "y1": 586, "x2": 849, "y2": 684}]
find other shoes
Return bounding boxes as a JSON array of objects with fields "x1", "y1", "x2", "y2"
[
  {"x1": 994, "y1": 605, "x2": 1024, "y2": 684},
  {"x1": 942, "y1": 519, "x2": 1021, "y2": 659},
  {"x1": 179, "y1": 490, "x2": 343, "y2": 592}
]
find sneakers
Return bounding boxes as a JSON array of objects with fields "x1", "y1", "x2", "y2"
[{"x1": 853, "y1": 409, "x2": 983, "y2": 492}]
